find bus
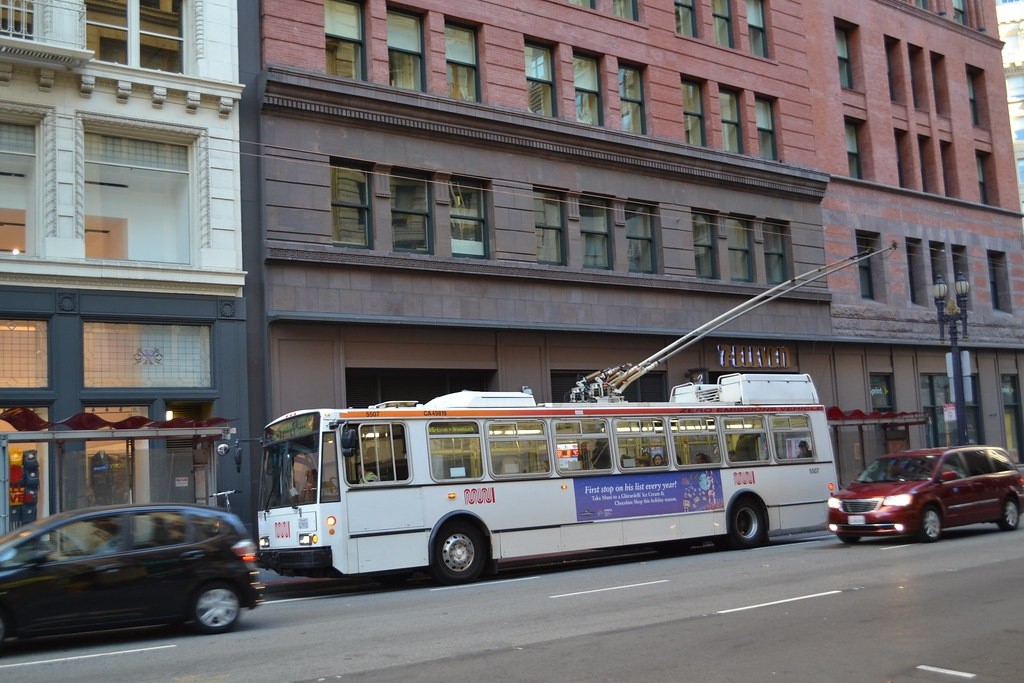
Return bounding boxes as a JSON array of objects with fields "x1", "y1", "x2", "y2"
[{"x1": 216, "y1": 243, "x2": 896, "y2": 584}]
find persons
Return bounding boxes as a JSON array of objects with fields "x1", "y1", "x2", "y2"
[
  {"x1": 695, "y1": 452, "x2": 712, "y2": 464},
  {"x1": 91, "y1": 450, "x2": 115, "y2": 505},
  {"x1": 796, "y1": 440, "x2": 812, "y2": 458},
  {"x1": 620, "y1": 452, "x2": 667, "y2": 467},
  {"x1": 353, "y1": 460, "x2": 378, "y2": 484},
  {"x1": 728, "y1": 450, "x2": 737, "y2": 462},
  {"x1": 304, "y1": 468, "x2": 319, "y2": 500}
]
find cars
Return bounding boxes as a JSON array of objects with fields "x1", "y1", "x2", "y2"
[
  {"x1": 1, "y1": 504, "x2": 262, "y2": 655},
  {"x1": 826, "y1": 445, "x2": 1024, "y2": 544}
]
